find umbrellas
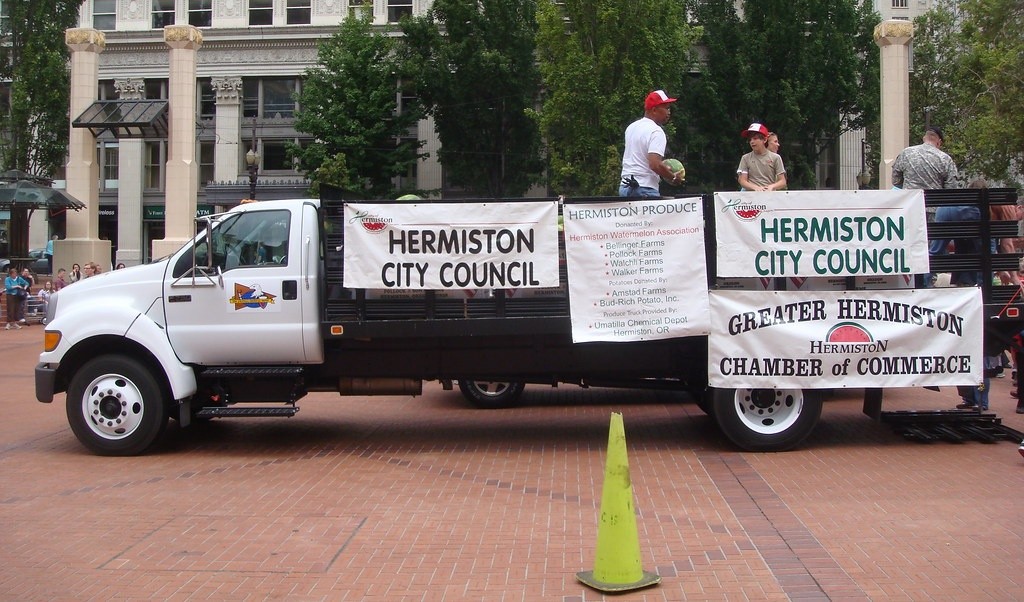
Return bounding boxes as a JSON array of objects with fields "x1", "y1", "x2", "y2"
[{"x1": 0, "y1": 169, "x2": 87, "y2": 255}]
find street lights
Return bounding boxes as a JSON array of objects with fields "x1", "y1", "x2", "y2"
[
  {"x1": 856, "y1": 138, "x2": 871, "y2": 189},
  {"x1": 246, "y1": 114, "x2": 260, "y2": 200}
]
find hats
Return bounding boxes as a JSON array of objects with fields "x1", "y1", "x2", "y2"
[
  {"x1": 645, "y1": 90, "x2": 676, "y2": 108},
  {"x1": 928, "y1": 127, "x2": 943, "y2": 140},
  {"x1": 741, "y1": 123, "x2": 768, "y2": 137}
]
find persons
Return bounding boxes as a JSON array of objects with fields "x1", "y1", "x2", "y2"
[
  {"x1": 46, "y1": 235, "x2": 58, "y2": 276},
  {"x1": 891, "y1": 129, "x2": 1024, "y2": 414},
  {"x1": 737, "y1": 124, "x2": 787, "y2": 191},
  {"x1": 254, "y1": 240, "x2": 267, "y2": 264},
  {"x1": 4, "y1": 261, "x2": 125, "y2": 330},
  {"x1": 618, "y1": 90, "x2": 685, "y2": 197}
]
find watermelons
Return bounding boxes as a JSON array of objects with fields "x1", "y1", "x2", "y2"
[
  {"x1": 557, "y1": 215, "x2": 566, "y2": 232},
  {"x1": 396, "y1": 194, "x2": 422, "y2": 201},
  {"x1": 662, "y1": 159, "x2": 684, "y2": 182},
  {"x1": 992, "y1": 277, "x2": 1001, "y2": 286}
]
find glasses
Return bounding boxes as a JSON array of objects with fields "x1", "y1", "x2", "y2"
[{"x1": 84, "y1": 267, "x2": 91, "y2": 269}]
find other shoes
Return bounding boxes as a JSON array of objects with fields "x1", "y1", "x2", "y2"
[
  {"x1": 996, "y1": 374, "x2": 1005, "y2": 377},
  {"x1": 18, "y1": 319, "x2": 25, "y2": 322},
  {"x1": 972, "y1": 404, "x2": 983, "y2": 410},
  {"x1": 956, "y1": 402, "x2": 971, "y2": 408},
  {"x1": 1005, "y1": 364, "x2": 1012, "y2": 368},
  {"x1": 1010, "y1": 367, "x2": 1024, "y2": 415}
]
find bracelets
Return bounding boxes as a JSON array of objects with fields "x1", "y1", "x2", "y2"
[{"x1": 671, "y1": 173, "x2": 676, "y2": 182}]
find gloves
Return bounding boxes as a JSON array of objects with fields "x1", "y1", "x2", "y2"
[{"x1": 622, "y1": 175, "x2": 639, "y2": 189}]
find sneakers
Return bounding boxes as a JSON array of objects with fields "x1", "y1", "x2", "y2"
[
  {"x1": 6, "y1": 323, "x2": 11, "y2": 329},
  {"x1": 11, "y1": 322, "x2": 21, "y2": 330}
]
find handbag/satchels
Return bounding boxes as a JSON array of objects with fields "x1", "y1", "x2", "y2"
[{"x1": 18, "y1": 276, "x2": 26, "y2": 300}]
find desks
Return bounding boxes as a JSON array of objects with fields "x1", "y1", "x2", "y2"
[{"x1": 9, "y1": 258, "x2": 38, "y2": 276}]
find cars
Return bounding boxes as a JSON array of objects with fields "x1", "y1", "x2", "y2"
[{"x1": 0, "y1": 249, "x2": 48, "y2": 273}]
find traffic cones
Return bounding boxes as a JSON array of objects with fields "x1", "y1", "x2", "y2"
[{"x1": 576, "y1": 408, "x2": 662, "y2": 592}]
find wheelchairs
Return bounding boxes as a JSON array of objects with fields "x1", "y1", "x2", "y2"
[{"x1": 24, "y1": 293, "x2": 47, "y2": 325}]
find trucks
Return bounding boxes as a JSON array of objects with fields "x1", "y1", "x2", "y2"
[{"x1": 34, "y1": 181, "x2": 1024, "y2": 457}]
